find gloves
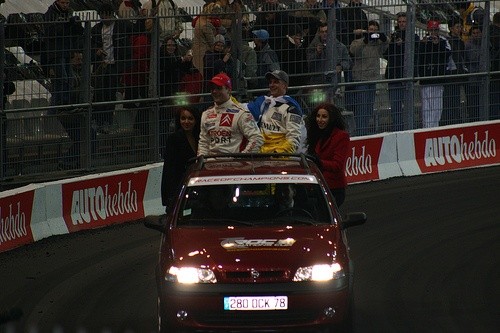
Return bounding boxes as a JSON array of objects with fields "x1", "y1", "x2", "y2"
[
  {"x1": 304, "y1": 153, "x2": 322, "y2": 168},
  {"x1": 377, "y1": 33, "x2": 386, "y2": 42},
  {"x1": 363, "y1": 32, "x2": 373, "y2": 43}
]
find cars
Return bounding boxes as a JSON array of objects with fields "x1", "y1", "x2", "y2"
[{"x1": 143, "y1": 153, "x2": 367, "y2": 333}]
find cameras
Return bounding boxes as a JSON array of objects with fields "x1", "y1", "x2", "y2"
[{"x1": 370, "y1": 34, "x2": 380, "y2": 39}]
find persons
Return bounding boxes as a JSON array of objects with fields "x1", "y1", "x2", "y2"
[
  {"x1": 262, "y1": 184, "x2": 314, "y2": 218},
  {"x1": 161, "y1": 106, "x2": 201, "y2": 213},
  {"x1": 43, "y1": 0, "x2": 500, "y2": 170},
  {"x1": 3, "y1": 72, "x2": 15, "y2": 160},
  {"x1": 196, "y1": 73, "x2": 265, "y2": 155},
  {"x1": 306, "y1": 104, "x2": 350, "y2": 207},
  {"x1": 240, "y1": 69, "x2": 304, "y2": 155}
]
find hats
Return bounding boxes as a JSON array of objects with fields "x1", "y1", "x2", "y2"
[
  {"x1": 252, "y1": 29, "x2": 269, "y2": 43},
  {"x1": 264, "y1": 70, "x2": 290, "y2": 85},
  {"x1": 214, "y1": 35, "x2": 226, "y2": 45},
  {"x1": 208, "y1": 74, "x2": 232, "y2": 89},
  {"x1": 427, "y1": 20, "x2": 439, "y2": 29},
  {"x1": 285, "y1": 23, "x2": 301, "y2": 35}
]
